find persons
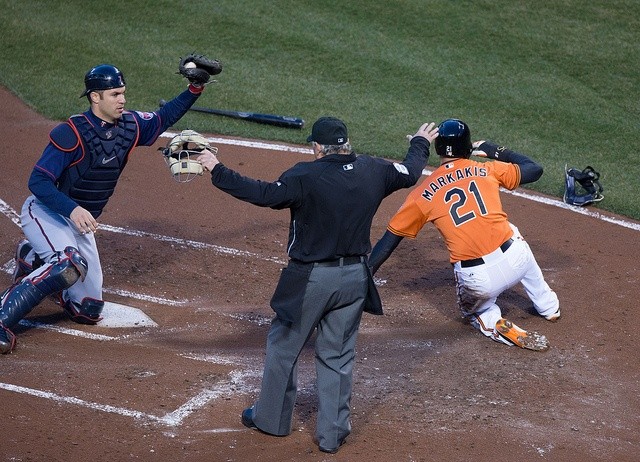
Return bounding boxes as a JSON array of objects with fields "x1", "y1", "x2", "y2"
[
  {"x1": 198, "y1": 116, "x2": 441, "y2": 452},
  {"x1": 367, "y1": 118, "x2": 560, "y2": 353},
  {"x1": 1, "y1": 51, "x2": 223, "y2": 355}
]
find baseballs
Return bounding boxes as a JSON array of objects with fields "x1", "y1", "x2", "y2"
[{"x1": 183, "y1": 61, "x2": 196, "y2": 70}]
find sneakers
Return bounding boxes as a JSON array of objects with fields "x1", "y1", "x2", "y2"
[
  {"x1": 12, "y1": 240, "x2": 39, "y2": 286},
  {"x1": 544, "y1": 308, "x2": 560, "y2": 322},
  {"x1": 242, "y1": 406, "x2": 259, "y2": 430},
  {"x1": 319, "y1": 438, "x2": 343, "y2": 454},
  {"x1": 495, "y1": 318, "x2": 550, "y2": 352},
  {"x1": 0, "y1": 325, "x2": 12, "y2": 354}
]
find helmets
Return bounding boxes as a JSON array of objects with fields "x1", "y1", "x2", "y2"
[
  {"x1": 435, "y1": 119, "x2": 471, "y2": 158},
  {"x1": 79, "y1": 64, "x2": 126, "y2": 99}
]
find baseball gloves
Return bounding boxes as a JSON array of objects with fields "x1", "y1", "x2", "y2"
[
  {"x1": 176, "y1": 50, "x2": 222, "y2": 86},
  {"x1": 563, "y1": 164, "x2": 604, "y2": 207}
]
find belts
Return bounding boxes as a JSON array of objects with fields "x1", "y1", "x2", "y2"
[
  {"x1": 318, "y1": 256, "x2": 367, "y2": 267},
  {"x1": 461, "y1": 239, "x2": 514, "y2": 268}
]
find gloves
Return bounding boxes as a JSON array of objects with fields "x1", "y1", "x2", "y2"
[{"x1": 471, "y1": 140, "x2": 512, "y2": 162}]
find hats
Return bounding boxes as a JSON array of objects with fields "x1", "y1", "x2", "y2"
[{"x1": 306, "y1": 117, "x2": 347, "y2": 145}]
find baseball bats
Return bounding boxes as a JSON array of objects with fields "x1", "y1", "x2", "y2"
[{"x1": 159, "y1": 98, "x2": 305, "y2": 129}]
